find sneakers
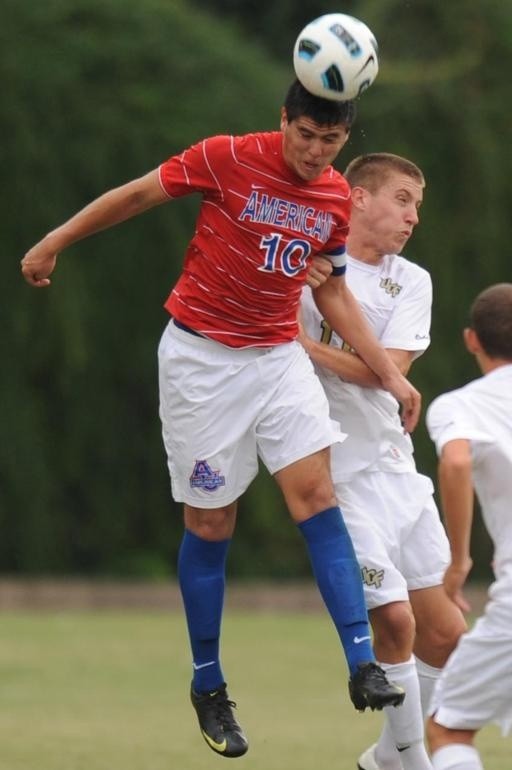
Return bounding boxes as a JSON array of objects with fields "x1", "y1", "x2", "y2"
[
  {"x1": 356, "y1": 743, "x2": 378, "y2": 770},
  {"x1": 190, "y1": 679, "x2": 249, "y2": 758},
  {"x1": 348, "y1": 662, "x2": 405, "y2": 712}
]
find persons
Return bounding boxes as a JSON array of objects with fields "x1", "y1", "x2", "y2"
[
  {"x1": 423, "y1": 282, "x2": 510, "y2": 768},
  {"x1": 298, "y1": 152, "x2": 466, "y2": 769},
  {"x1": 17, "y1": 77, "x2": 423, "y2": 758}
]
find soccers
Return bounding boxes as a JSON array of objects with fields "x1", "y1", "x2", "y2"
[{"x1": 293, "y1": 13, "x2": 379, "y2": 102}]
